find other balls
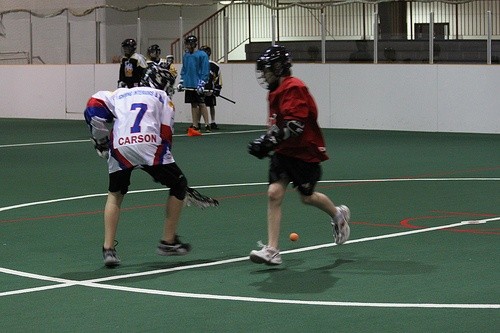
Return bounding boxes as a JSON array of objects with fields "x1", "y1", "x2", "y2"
[{"x1": 288, "y1": 232, "x2": 299, "y2": 242}]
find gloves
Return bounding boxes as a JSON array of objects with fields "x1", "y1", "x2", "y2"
[
  {"x1": 197, "y1": 80, "x2": 206, "y2": 95},
  {"x1": 177, "y1": 80, "x2": 184, "y2": 92},
  {"x1": 90, "y1": 136, "x2": 109, "y2": 159},
  {"x1": 247, "y1": 120, "x2": 305, "y2": 161},
  {"x1": 213, "y1": 82, "x2": 222, "y2": 97}
]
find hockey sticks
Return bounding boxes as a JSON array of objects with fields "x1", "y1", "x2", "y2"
[
  {"x1": 215, "y1": 93, "x2": 236, "y2": 104},
  {"x1": 99, "y1": 143, "x2": 220, "y2": 209},
  {"x1": 172, "y1": 87, "x2": 214, "y2": 93},
  {"x1": 248, "y1": 144, "x2": 271, "y2": 159}
]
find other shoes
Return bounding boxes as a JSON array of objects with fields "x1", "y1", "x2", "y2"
[
  {"x1": 211, "y1": 122, "x2": 219, "y2": 130},
  {"x1": 204, "y1": 124, "x2": 211, "y2": 132},
  {"x1": 186, "y1": 125, "x2": 201, "y2": 132}
]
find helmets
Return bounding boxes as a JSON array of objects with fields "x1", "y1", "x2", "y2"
[
  {"x1": 200, "y1": 45, "x2": 211, "y2": 57},
  {"x1": 146, "y1": 45, "x2": 161, "y2": 54},
  {"x1": 121, "y1": 39, "x2": 137, "y2": 51},
  {"x1": 256, "y1": 45, "x2": 292, "y2": 77},
  {"x1": 184, "y1": 35, "x2": 198, "y2": 48}
]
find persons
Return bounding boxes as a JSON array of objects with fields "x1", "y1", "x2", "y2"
[
  {"x1": 248, "y1": 46, "x2": 350, "y2": 267},
  {"x1": 118, "y1": 36, "x2": 222, "y2": 132},
  {"x1": 84, "y1": 68, "x2": 190, "y2": 266}
]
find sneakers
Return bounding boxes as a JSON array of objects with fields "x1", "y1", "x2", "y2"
[
  {"x1": 250, "y1": 240, "x2": 283, "y2": 266},
  {"x1": 155, "y1": 233, "x2": 192, "y2": 256},
  {"x1": 331, "y1": 204, "x2": 351, "y2": 245},
  {"x1": 103, "y1": 240, "x2": 120, "y2": 266}
]
